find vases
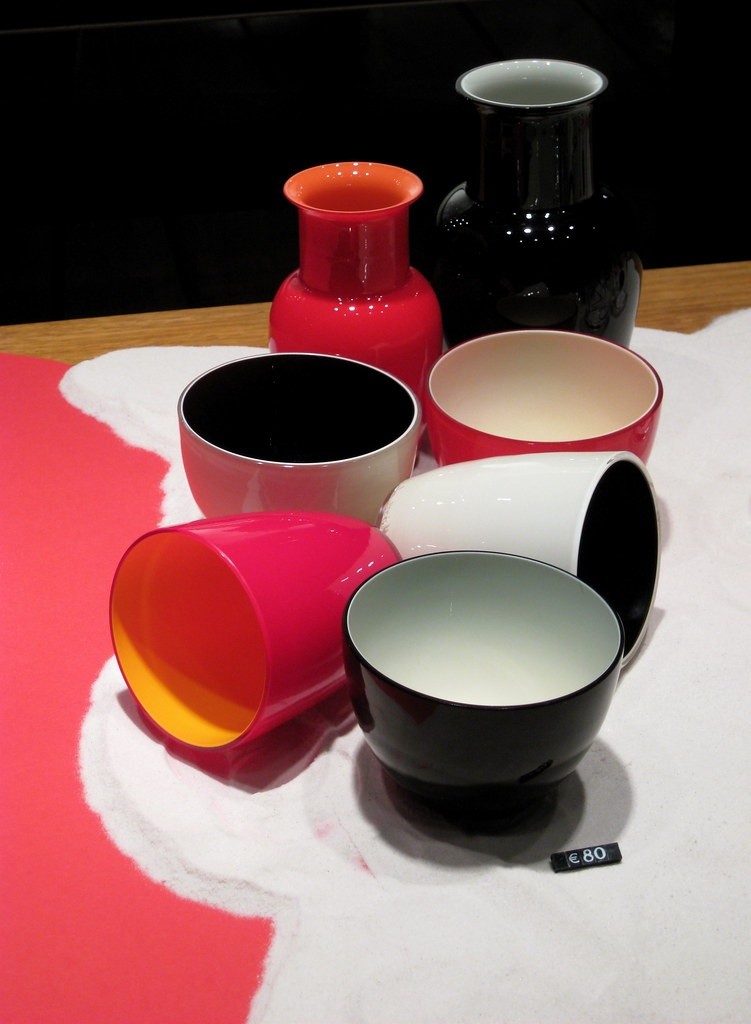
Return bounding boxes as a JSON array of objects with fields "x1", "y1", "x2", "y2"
[
  {"x1": 438, "y1": 54, "x2": 640, "y2": 351},
  {"x1": 267, "y1": 160, "x2": 445, "y2": 449}
]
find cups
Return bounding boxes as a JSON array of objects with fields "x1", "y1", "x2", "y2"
[
  {"x1": 381, "y1": 450, "x2": 660, "y2": 671},
  {"x1": 176, "y1": 352, "x2": 423, "y2": 534},
  {"x1": 108, "y1": 509, "x2": 407, "y2": 750},
  {"x1": 339, "y1": 550, "x2": 626, "y2": 807},
  {"x1": 426, "y1": 328, "x2": 665, "y2": 476}
]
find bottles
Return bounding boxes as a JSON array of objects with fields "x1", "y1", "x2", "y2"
[
  {"x1": 269, "y1": 161, "x2": 442, "y2": 463},
  {"x1": 426, "y1": 57, "x2": 641, "y2": 350}
]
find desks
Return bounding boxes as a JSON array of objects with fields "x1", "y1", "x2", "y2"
[{"x1": 1, "y1": 261, "x2": 751, "y2": 1024}]
